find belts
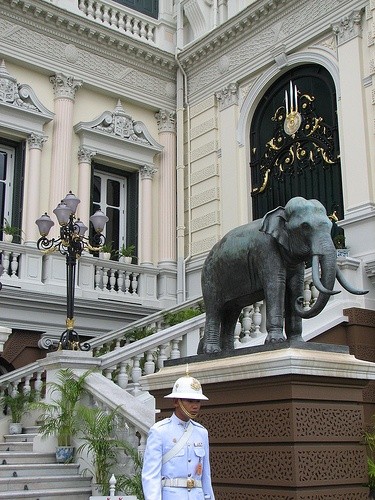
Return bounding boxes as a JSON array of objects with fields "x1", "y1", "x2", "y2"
[{"x1": 164, "y1": 478, "x2": 203, "y2": 489}]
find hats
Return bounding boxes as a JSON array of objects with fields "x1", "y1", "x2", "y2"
[{"x1": 163, "y1": 362, "x2": 209, "y2": 401}]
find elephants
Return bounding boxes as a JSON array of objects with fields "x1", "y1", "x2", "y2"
[{"x1": 199, "y1": 196, "x2": 369, "y2": 352}]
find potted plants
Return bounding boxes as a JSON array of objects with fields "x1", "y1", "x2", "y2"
[
  {"x1": 101, "y1": 241, "x2": 116, "y2": 260},
  {"x1": 0, "y1": 368, "x2": 138, "y2": 496},
  {"x1": 0, "y1": 218, "x2": 25, "y2": 243},
  {"x1": 116, "y1": 243, "x2": 138, "y2": 264}
]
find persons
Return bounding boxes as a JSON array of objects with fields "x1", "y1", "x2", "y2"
[{"x1": 142, "y1": 377, "x2": 215, "y2": 500}]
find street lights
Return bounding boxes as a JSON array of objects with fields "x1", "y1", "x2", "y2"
[{"x1": 35, "y1": 190, "x2": 110, "y2": 351}]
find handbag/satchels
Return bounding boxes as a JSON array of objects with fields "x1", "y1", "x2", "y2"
[{"x1": 161, "y1": 476, "x2": 166, "y2": 488}]
